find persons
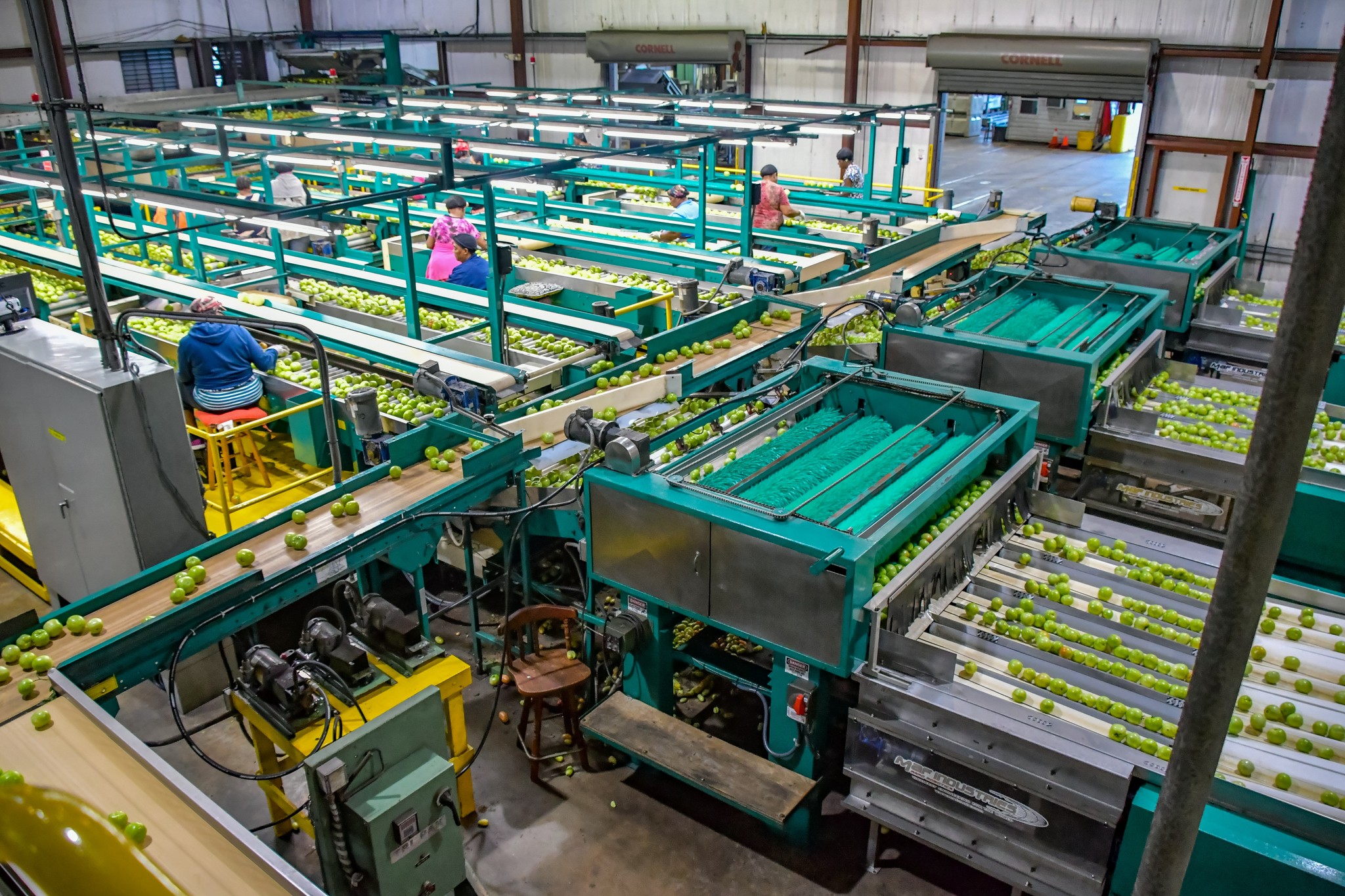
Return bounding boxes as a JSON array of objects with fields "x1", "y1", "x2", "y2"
[
  {"x1": 265, "y1": 162, "x2": 316, "y2": 253},
  {"x1": 527, "y1": 91, "x2": 539, "y2": 101},
  {"x1": 572, "y1": 131, "x2": 595, "y2": 147},
  {"x1": 175, "y1": 296, "x2": 290, "y2": 451},
  {"x1": 152, "y1": 174, "x2": 199, "y2": 232},
  {"x1": 441, "y1": 233, "x2": 489, "y2": 291},
  {"x1": 398, "y1": 133, "x2": 491, "y2": 214},
  {"x1": 822, "y1": 146, "x2": 864, "y2": 199},
  {"x1": 227, "y1": 175, "x2": 267, "y2": 238},
  {"x1": 424, "y1": 195, "x2": 489, "y2": 282},
  {"x1": 751, "y1": 164, "x2": 805, "y2": 251},
  {"x1": 517, "y1": 127, "x2": 530, "y2": 141},
  {"x1": 649, "y1": 183, "x2": 699, "y2": 241}
]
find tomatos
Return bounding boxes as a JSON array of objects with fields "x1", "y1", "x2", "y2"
[{"x1": 0, "y1": 107, "x2": 1345, "y2": 844}]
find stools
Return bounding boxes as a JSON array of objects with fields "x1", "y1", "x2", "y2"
[{"x1": 199, "y1": 414, "x2": 273, "y2": 502}]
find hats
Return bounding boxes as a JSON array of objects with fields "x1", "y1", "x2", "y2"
[
  {"x1": 189, "y1": 296, "x2": 227, "y2": 313},
  {"x1": 668, "y1": 184, "x2": 689, "y2": 198},
  {"x1": 451, "y1": 233, "x2": 478, "y2": 251}
]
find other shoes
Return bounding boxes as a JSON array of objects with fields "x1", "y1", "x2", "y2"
[{"x1": 189, "y1": 434, "x2": 206, "y2": 450}]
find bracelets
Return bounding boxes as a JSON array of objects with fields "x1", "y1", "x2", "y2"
[{"x1": 657, "y1": 232, "x2": 662, "y2": 240}]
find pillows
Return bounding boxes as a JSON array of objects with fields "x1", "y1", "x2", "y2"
[
  {"x1": 194, "y1": 407, "x2": 273, "y2": 431},
  {"x1": 509, "y1": 281, "x2": 566, "y2": 300}
]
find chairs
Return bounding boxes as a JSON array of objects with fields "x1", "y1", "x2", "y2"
[
  {"x1": 981, "y1": 117, "x2": 995, "y2": 142},
  {"x1": 499, "y1": 604, "x2": 594, "y2": 782}
]
